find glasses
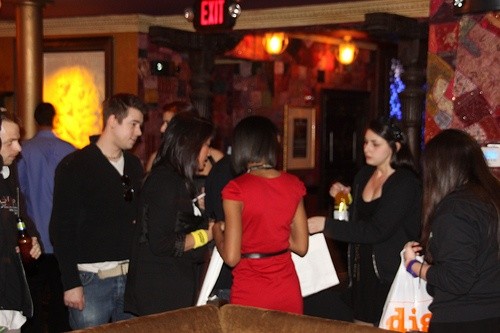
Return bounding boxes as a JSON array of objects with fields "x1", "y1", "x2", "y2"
[{"x1": 121, "y1": 174, "x2": 134, "y2": 203}]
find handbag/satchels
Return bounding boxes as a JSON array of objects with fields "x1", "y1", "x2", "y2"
[
  {"x1": 380, "y1": 248, "x2": 433, "y2": 332},
  {"x1": 191, "y1": 246, "x2": 224, "y2": 306},
  {"x1": 292, "y1": 233, "x2": 340, "y2": 297}
]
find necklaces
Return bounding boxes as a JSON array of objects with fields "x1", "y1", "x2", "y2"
[{"x1": 247, "y1": 163, "x2": 273, "y2": 171}]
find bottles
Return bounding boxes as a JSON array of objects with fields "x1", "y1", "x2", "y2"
[
  {"x1": 16, "y1": 215, "x2": 33, "y2": 260},
  {"x1": 333, "y1": 187, "x2": 350, "y2": 221}
]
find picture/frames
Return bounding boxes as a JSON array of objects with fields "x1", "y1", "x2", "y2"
[
  {"x1": 283, "y1": 105, "x2": 317, "y2": 171},
  {"x1": 41, "y1": 35, "x2": 115, "y2": 150}
]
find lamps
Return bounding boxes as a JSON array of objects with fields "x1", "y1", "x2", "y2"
[
  {"x1": 335, "y1": 35, "x2": 359, "y2": 65},
  {"x1": 184, "y1": 0, "x2": 242, "y2": 31},
  {"x1": 262, "y1": 31, "x2": 289, "y2": 55}
]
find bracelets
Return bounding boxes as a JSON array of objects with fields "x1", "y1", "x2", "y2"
[
  {"x1": 419, "y1": 263, "x2": 423, "y2": 278},
  {"x1": 190, "y1": 229, "x2": 209, "y2": 249}
]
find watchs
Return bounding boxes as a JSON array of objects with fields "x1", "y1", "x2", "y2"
[{"x1": 406, "y1": 259, "x2": 421, "y2": 279}]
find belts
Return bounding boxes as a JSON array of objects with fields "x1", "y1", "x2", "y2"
[{"x1": 96, "y1": 263, "x2": 129, "y2": 279}]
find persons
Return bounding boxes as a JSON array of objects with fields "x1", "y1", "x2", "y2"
[{"x1": 0, "y1": 93, "x2": 500, "y2": 333}]
